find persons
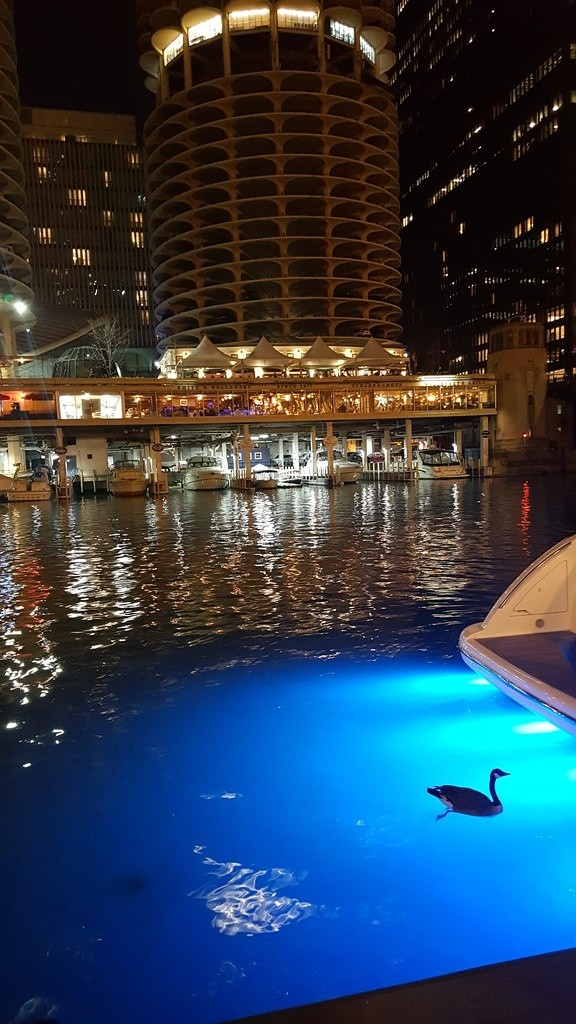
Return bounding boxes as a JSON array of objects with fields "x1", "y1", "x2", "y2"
[
  {"x1": 163, "y1": 404, "x2": 188, "y2": 417},
  {"x1": 199, "y1": 404, "x2": 216, "y2": 416}
]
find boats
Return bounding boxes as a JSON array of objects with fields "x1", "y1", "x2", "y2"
[
  {"x1": 458, "y1": 534, "x2": 576, "y2": 734},
  {"x1": 182, "y1": 456, "x2": 229, "y2": 489},
  {"x1": 251, "y1": 464, "x2": 278, "y2": 489},
  {"x1": 390, "y1": 448, "x2": 470, "y2": 479},
  {"x1": 108, "y1": 459, "x2": 148, "y2": 496},
  {"x1": 306, "y1": 449, "x2": 363, "y2": 484}
]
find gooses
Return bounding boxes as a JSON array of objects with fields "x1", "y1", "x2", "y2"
[{"x1": 427, "y1": 768, "x2": 511, "y2": 821}]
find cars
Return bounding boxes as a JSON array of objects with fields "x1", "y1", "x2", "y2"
[
  {"x1": 274, "y1": 454, "x2": 293, "y2": 465},
  {"x1": 373, "y1": 452, "x2": 384, "y2": 457},
  {"x1": 357, "y1": 450, "x2": 363, "y2": 456},
  {"x1": 299, "y1": 452, "x2": 309, "y2": 460}
]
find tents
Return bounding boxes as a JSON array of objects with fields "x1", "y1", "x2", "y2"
[
  {"x1": 233, "y1": 336, "x2": 302, "y2": 378},
  {"x1": 288, "y1": 338, "x2": 356, "y2": 376},
  {"x1": 176, "y1": 334, "x2": 244, "y2": 378},
  {"x1": 339, "y1": 337, "x2": 411, "y2": 375}
]
